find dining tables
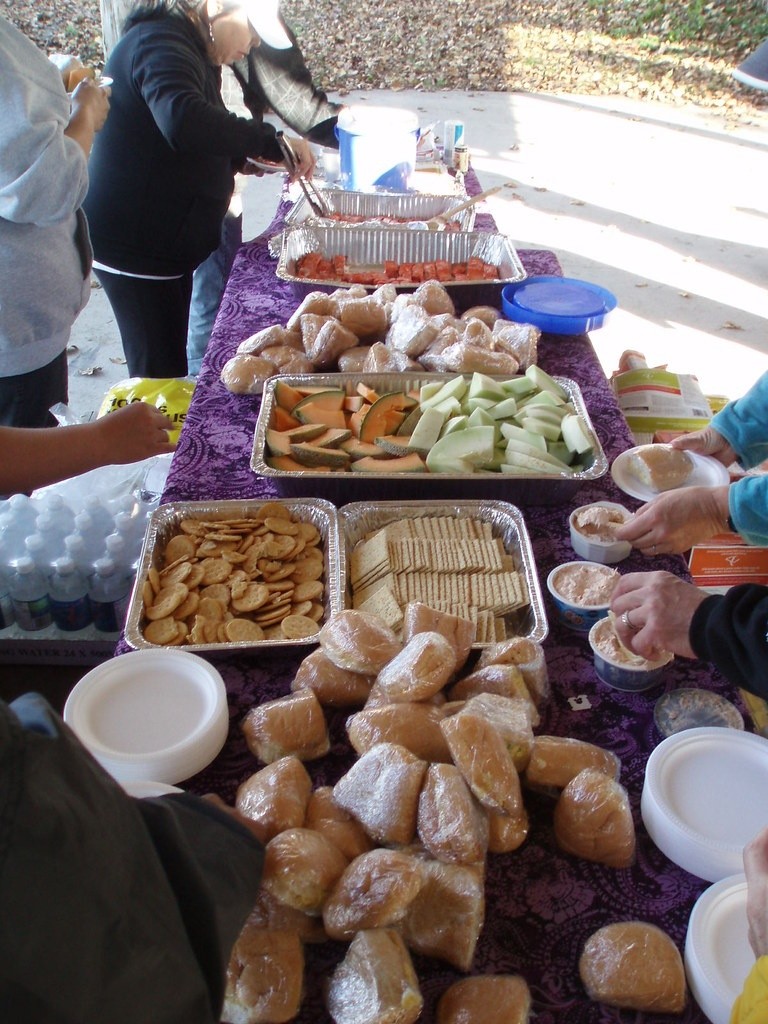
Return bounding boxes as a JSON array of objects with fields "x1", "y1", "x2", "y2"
[{"x1": 116, "y1": 149, "x2": 763, "y2": 1024}]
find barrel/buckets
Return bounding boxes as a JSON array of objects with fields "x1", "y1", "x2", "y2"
[{"x1": 335, "y1": 104, "x2": 418, "y2": 195}]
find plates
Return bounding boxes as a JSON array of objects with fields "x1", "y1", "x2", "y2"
[
  {"x1": 63, "y1": 648, "x2": 230, "y2": 786},
  {"x1": 67, "y1": 77, "x2": 113, "y2": 96},
  {"x1": 247, "y1": 157, "x2": 287, "y2": 173},
  {"x1": 642, "y1": 728, "x2": 767, "y2": 1023},
  {"x1": 612, "y1": 445, "x2": 732, "y2": 502}
]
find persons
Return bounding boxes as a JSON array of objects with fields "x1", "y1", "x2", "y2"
[
  {"x1": 0, "y1": 399, "x2": 177, "y2": 505},
  {"x1": 726, "y1": 825, "x2": 767, "y2": 1023},
  {"x1": 75, "y1": 0, "x2": 316, "y2": 380},
  {"x1": 608, "y1": 570, "x2": 767, "y2": 698},
  {"x1": 0, "y1": 21, "x2": 113, "y2": 425},
  {"x1": 616, "y1": 366, "x2": 768, "y2": 559},
  {"x1": 1, "y1": 692, "x2": 267, "y2": 1023},
  {"x1": 187, "y1": 13, "x2": 347, "y2": 370}
]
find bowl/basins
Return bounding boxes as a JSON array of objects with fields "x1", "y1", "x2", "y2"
[
  {"x1": 547, "y1": 560, "x2": 622, "y2": 631},
  {"x1": 587, "y1": 615, "x2": 674, "y2": 691},
  {"x1": 568, "y1": 502, "x2": 631, "y2": 565}
]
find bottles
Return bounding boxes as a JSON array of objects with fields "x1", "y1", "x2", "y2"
[
  {"x1": 455, "y1": 144, "x2": 469, "y2": 177},
  {"x1": 444, "y1": 119, "x2": 465, "y2": 166},
  {"x1": 1, "y1": 495, "x2": 145, "y2": 645}
]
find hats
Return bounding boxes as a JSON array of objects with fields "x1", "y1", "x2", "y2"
[{"x1": 239, "y1": 0, "x2": 293, "y2": 49}]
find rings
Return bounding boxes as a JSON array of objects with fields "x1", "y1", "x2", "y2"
[
  {"x1": 621, "y1": 610, "x2": 641, "y2": 634},
  {"x1": 652, "y1": 545, "x2": 659, "y2": 556}
]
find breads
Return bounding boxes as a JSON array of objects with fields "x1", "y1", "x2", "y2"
[
  {"x1": 221, "y1": 280, "x2": 541, "y2": 394},
  {"x1": 218, "y1": 604, "x2": 688, "y2": 1024},
  {"x1": 628, "y1": 445, "x2": 694, "y2": 493},
  {"x1": 47, "y1": 53, "x2": 82, "y2": 89}
]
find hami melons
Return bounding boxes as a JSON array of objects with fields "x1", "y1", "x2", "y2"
[
  {"x1": 265, "y1": 380, "x2": 427, "y2": 475},
  {"x1": 67, "y1": 68, "x2": 96, "y2": 93}
]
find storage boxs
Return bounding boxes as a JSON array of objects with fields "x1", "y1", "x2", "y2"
[{"x1": 651, "y1": 426, "x2": 767, "y2": 592}]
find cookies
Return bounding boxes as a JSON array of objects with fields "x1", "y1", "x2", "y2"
[
  {"x1": 349, "y1": 516, "x2": 532, "y2": 646},
  {"x1": 141, "y1": 502, "x2": 326, "y2": 648}
]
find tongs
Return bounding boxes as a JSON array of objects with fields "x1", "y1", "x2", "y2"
[{"x1": 275, "y1": 130, "x2": 329, "y2": 218}]
